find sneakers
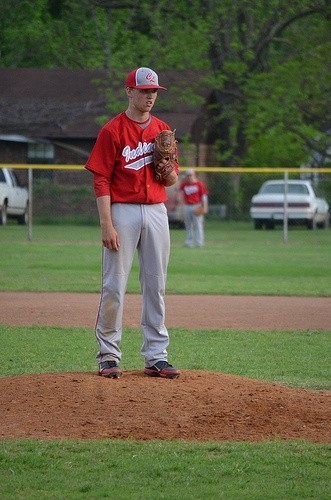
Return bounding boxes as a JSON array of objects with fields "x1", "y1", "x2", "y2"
[
  {"x1": 143, "y1": 361, "x2": 181, "y2": 377},
  {"x1": 99, "y1": 360, "x2": 122, "y2": 378}
]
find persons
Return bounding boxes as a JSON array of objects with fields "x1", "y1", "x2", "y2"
[
  {"x1": 180, "y1": 169, "x2": 208, "y2": 247},
  {"x1": 85, "y1": 67, "x2": 180, "y2": 379}
]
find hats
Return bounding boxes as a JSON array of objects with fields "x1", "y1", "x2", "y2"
[
  {"x1": 186, "y1": 169, "x2": 196, "y2": 177},
  {"x1": 126, "y1": 67, "x2": 167, "y2": 92}
]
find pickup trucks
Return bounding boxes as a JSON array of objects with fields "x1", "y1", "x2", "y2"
[{"x1": 0, "y1": 166, "x2": 30, "y2": 227}]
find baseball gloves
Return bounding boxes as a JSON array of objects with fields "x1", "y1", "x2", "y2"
[
  {"x1": 192, "y1": 206, "x2": 207, "y2": 215},
  {"x1": 151, "y1": 130, "x2": 177, "y2": 182}
]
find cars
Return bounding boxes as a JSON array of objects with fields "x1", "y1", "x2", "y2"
[
  {"x1": 250, "y1": 179, "x2": 329, "y2": 231},
  {"x1": 165, "y1": 180, "x2": 188, "y2": 229}
]
